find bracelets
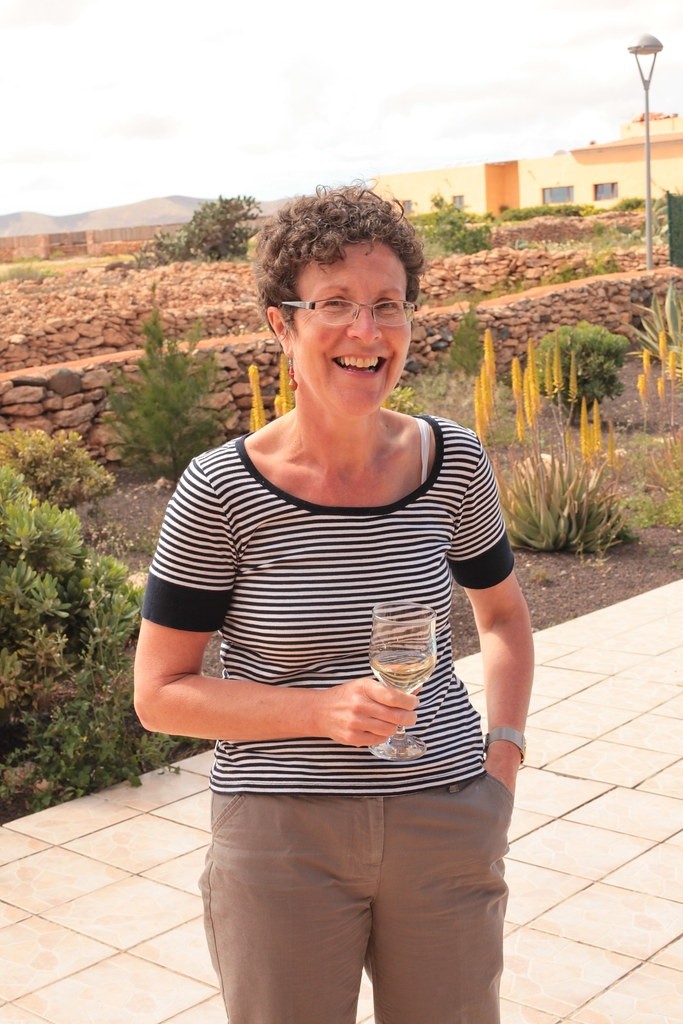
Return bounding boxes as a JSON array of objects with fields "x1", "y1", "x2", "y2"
[{"x1": 483, "y1": 725, "x2": 527, "y2": 762}]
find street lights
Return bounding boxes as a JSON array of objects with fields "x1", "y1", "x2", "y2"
[{"x1": 628, "y1": 35, "x2": 663, "y2": 269}]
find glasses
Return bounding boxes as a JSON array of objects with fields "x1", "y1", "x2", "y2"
[{"x1": 281, "y1": 299, "x2": 414, "y2": 327}]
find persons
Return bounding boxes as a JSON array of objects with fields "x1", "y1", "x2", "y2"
[{"x1": 134, "y1": 179, "x2": 534, "y2": 1024}]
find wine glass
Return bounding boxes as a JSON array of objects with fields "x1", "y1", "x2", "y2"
[{"x1": 369, "y1": 602, "x2": 437, "y2": 761}]
